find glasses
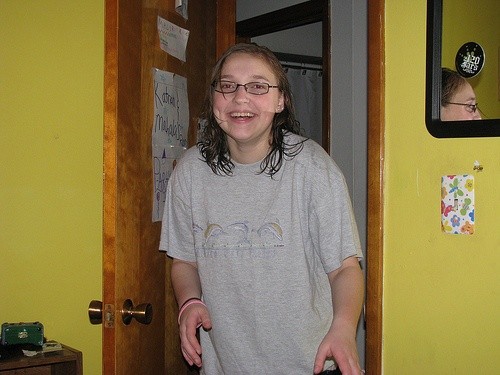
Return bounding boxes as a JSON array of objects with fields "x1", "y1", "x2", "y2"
[
  {"x1": 211, "y1": 80, "x2": 280, "y2": 95},
  {"x1": 449, "y1": 100, "x2": 479, "y2": 113}
]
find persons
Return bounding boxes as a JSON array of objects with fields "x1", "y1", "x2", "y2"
[
  {"x1": 440, "y1": 66, "x2": 482, "y2": 120},
  {"x1": 159, "y1": 42, "x2": 367, "y2": 375}
]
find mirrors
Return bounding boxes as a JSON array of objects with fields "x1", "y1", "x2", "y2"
[{"x1": 425, "y1": 0, "x2": 500, "y2": 139}]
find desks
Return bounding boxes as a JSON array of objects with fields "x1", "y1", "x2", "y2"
[{"x1": 0, "y1": 339, "x2": 84, "y2": 375}]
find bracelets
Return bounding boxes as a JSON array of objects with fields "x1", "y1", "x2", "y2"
[{"x1": 178, "y1": 296, "x2": 208, "y2": 328}]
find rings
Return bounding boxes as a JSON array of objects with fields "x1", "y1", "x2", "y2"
[{"x1": 360, "y1": 368, "x2": 366, "y2": 373}]
